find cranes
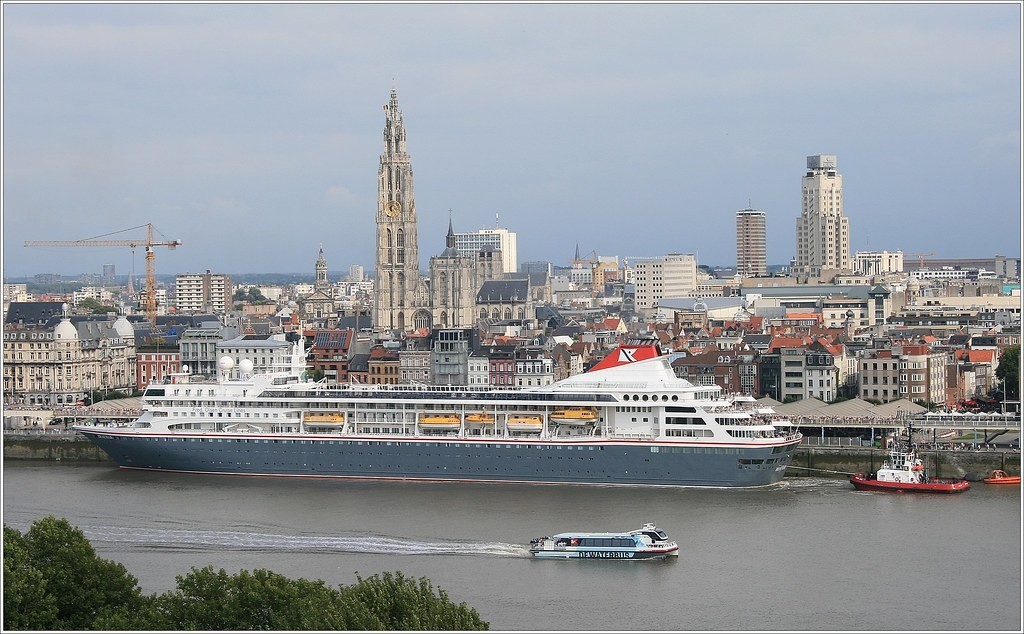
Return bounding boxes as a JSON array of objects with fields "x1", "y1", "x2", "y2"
[
  {"x1": 570, "y1": 244, "x2": 598, "y2": 268},
  {"x1": 24, "y1": 223, "x2": 182, "y2": 325},
  {"x1": 904, "y1": 253, "x2": 934, "y2": 268}
]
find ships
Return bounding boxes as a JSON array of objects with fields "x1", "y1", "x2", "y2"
[{"x1": 71, "y1": 320, "x2": 803, "y2": 488}]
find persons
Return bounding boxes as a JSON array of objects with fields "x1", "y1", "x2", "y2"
[
  {"x1": 530, "y1": 536, "x2": 552, "y2": 546},
  {"x1": 929, "y1": 477, "x2": 960, "y2": 484},
  {"x1": 890, "y1": 438, "x2": 1016, "y2": 452}
]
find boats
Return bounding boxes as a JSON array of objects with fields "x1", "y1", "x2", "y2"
[
  {"x1": 850, "y1": 449, "x2": 970, "y2": 494},
  {"x1": 303, "y1": 414, "x2": 344, "y2": 426},
  {"x1": 506, "y1": 418, "x2": 543, "y2": 432},
  {"x1": 528, "y1": 523, "x2": 680, "y2": 560},
  {"x1": 984, "y1": 470, "x2": 1021, "y2": 484},
  {"x1": 418, "y1": 416, "x2": 460, "y2": 429},
  {"x1": 550, "y1": 410, "x2": 599, "y2": 425},
  {"x1": 465, "y1": 412, "x2": 494, "y2": 424}
]
